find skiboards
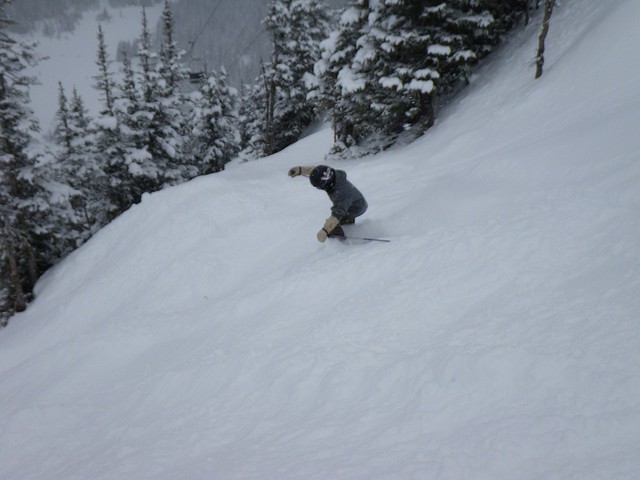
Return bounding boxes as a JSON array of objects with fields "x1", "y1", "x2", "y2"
[{"x1": 318, "y1": 230, "x2": 393, "y2": 245}]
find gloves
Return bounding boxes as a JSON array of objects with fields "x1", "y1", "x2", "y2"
[
  {"x1": 316, "y1": 216, "x2": 339, "y2": 242},
  {"x1": 288, "y1": 166, "x2": 315, "y2": 179}
]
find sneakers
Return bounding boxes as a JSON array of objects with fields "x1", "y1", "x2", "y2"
[
  {"x1": 340, "y1": 216, "x2": 355, "y2": 225},
  {"x1": 328, "y1": 226, "x2": 344, "y2": 237}
]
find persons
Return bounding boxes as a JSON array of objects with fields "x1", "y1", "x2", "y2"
[{"x1": 287, "y1": 165, "x2": 368, "y2": 243}]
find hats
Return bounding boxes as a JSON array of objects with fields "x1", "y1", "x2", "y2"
[{"x1": 309, "y1": 165, "x2": 335, "y2": 190}]
